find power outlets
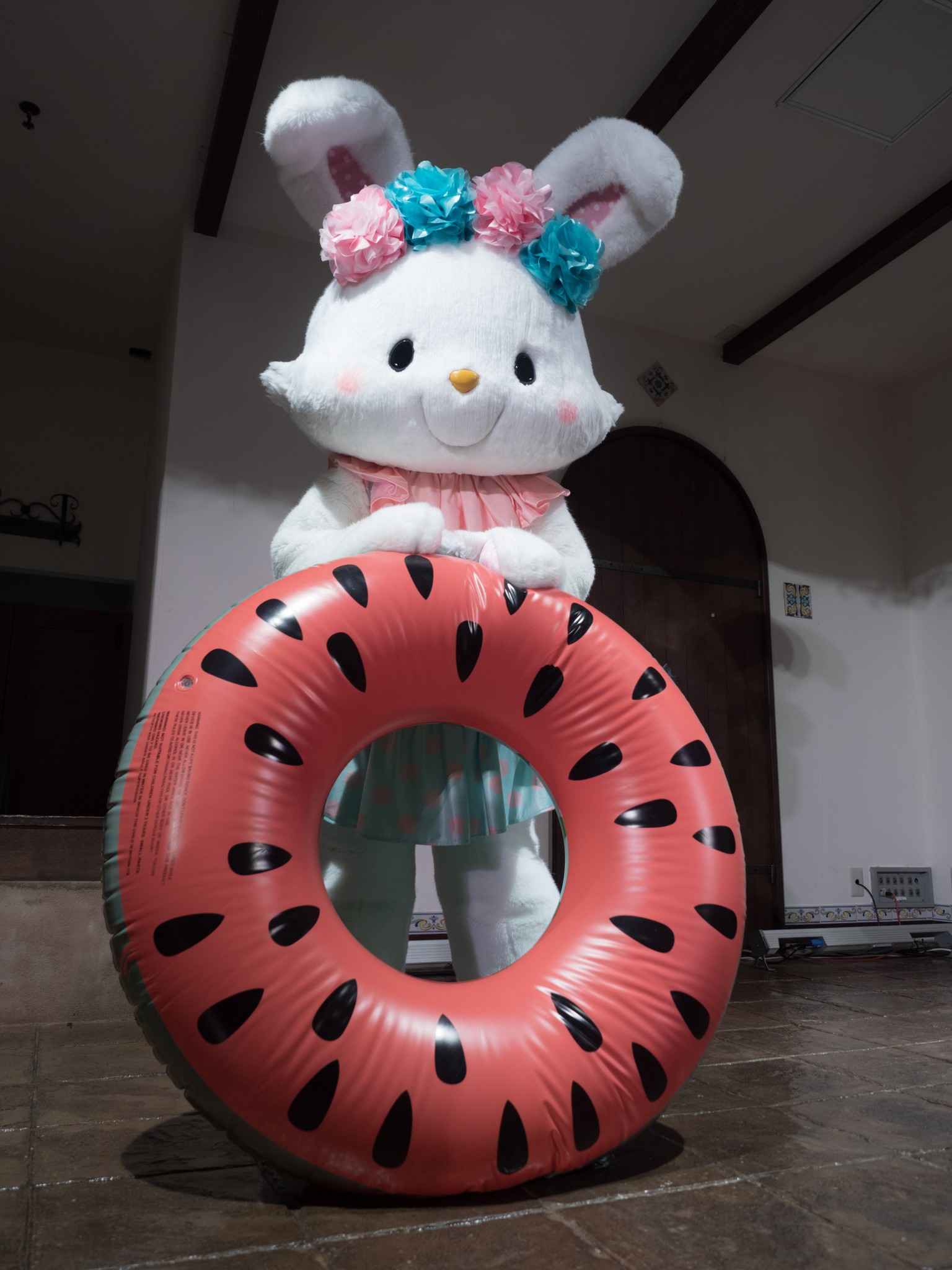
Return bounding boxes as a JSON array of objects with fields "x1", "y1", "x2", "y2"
[{"x1": 850, "y1": 867, "x2": 864, "y2": 896}]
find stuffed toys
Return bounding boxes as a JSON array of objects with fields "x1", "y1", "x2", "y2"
[{"x1": 262, "y1": 73, "x2": 682, "y2": 985}]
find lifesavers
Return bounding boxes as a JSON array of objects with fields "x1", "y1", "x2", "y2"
[{"x1": 96, "y1": 547, "x2": 754, "y2": 1204}]
